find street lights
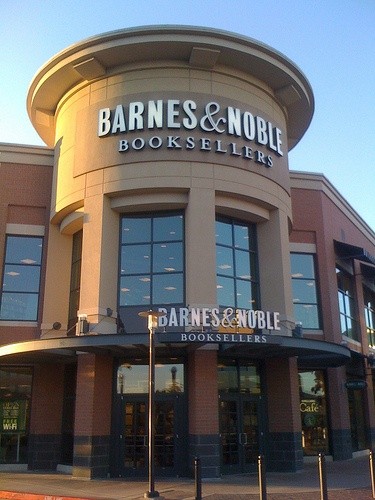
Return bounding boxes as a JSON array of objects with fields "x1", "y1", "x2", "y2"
[{"x1": 138, "y1": 309, "x2": 168, "y2": 499}]
